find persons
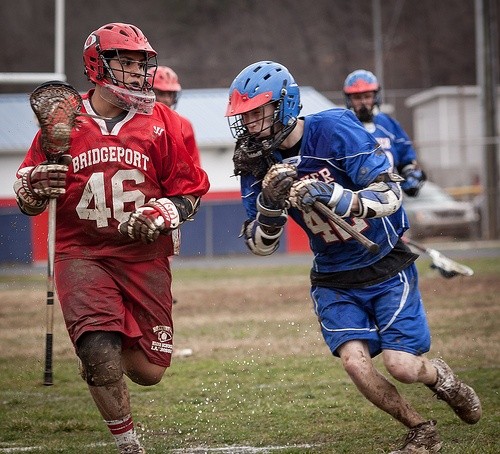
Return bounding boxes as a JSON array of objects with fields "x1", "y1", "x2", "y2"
[
  {"x1": 343, "y1": 69, "x2": 427, "y2": 197},
  {"x1": 224, "y1": 61, "x2": 481, "y2": 454},
  {"x1": 146, "y1": 65, "x2": 181, "y2": 108},
  {"x1": 13, "y1": 22, "x2": 210, "y2": 454}
]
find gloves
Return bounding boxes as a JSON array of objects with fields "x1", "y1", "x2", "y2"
[
  {"x1": 400, "y1": 165, "x2": 427, "y2": 198},
  {"x1": 256, "y1": 164, "x2": 298, "y2": 217},
  {"x1": 22, "y1": 154, "x2": 72, "y2": 198},
  {"x1": 118, "y1": 198, "x2": 179, "y2": 243},
  {"x1": 289, "y1": 179, "x2": 354, "y2": 218}
]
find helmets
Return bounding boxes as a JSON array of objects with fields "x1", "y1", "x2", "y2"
[
  {"x1": 344, "y1": 70, "x2": 380, "y2": 122},
  {"x1": 225, "y1": 61, "x2": 303, "y2": 156},
  {"x1": 83, "y1": 22, "x2": 158, "y2": 115},
  {"x1": 147, "y1": 66, "x2": 182, "y2": 91}
]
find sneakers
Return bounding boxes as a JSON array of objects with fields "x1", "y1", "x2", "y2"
[
  {"x1": 387, "y1": 420, "x2": 443, "y2": 454},
  {"x1": 425, "y1": 358, "x2": 481, "y2": 424}
]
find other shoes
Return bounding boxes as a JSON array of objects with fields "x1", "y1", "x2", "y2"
[{"x1": 120, "y1": 443, "x2": 145, "y2": 454}]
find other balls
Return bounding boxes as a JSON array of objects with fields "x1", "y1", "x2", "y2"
[{"x1": 51, "y1": 123, "x2": 71, "y2": 141}]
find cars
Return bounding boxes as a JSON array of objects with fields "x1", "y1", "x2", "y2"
[{"x1": 401, "y1": 179, "x2": 481, "y2": 242}]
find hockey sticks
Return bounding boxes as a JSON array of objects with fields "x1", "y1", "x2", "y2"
[
  {"x1": 399, "y1": 235, "x2": 474, "y2": 278},
  {"x1": 29, "y1": 79, "x2": 82, "y2": 387},
  {"x1": 234, "y1": 141, "x2": 381, "y2": 255}
]
